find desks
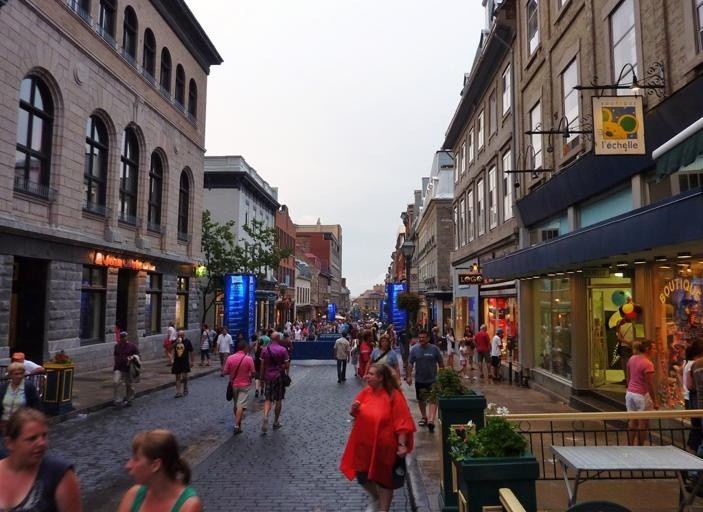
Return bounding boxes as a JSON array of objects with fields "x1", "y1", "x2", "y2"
[{"x1": 546, "y1": 444, "x2": 703, "y2": 512}]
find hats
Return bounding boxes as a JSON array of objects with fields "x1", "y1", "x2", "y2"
[
  {"x1": 496, "y1": 329, "x2": 503, "y2": 334},
  {"x1": 12, "y1": 352, "x2": 24, "y2": 359}
]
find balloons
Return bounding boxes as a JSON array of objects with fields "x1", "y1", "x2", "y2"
[{"x1": 611, "y1": 290, "x2": 642, "y2": 319}]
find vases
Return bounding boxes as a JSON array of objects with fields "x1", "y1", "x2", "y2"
[{"x1": 454, "y1": 457, "x2": 539, "y2": 512}]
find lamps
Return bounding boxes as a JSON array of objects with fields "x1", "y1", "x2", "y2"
[{"x1": 616, "y1": 63, "x2": 642, "y2": 91}]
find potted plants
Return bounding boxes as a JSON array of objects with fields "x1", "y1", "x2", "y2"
[{"x1": 425, "y1": 367, "x2": 487, "y2": 512}]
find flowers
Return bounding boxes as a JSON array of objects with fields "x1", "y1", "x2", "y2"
[
  {"x1": 50, "y1": 349, "x2": 73, "y2": 363},
  {"x1": 449, "y1": 403, "x2": 529, "y2": 458}
]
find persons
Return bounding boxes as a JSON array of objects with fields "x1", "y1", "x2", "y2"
[
  {"x1": 340, "y1": 363, "x2": 416, "y2": 511},
  {"x1": 254, "y1": 338, "x2": 266, "y2": 398},
  {"x1": 116, "y1": 427, "x2": 204, "y2": 512},
  {"x1": 200, "y1": 317, "x2": 318, "y2": 376},
  {"x1": 625, "y1": 341, "x2": 661, "y2": 446},
  {"x1": 430, "y1": 323, "x2": 504, "y2": 380},
  {"x1": 616, "y1": 306, "x2": 634, "y2": 387},
  {"x1": 112, "y1": 331, "x2": 140, "y2": 410},
  {"x1": 223, "y1": 341, "x2": 256, "y2": 435},
  {"x1": 259, "y1": 331, "x2": 290, "y2": 432},
  {"x1": 0, "y1": 349, "x2": 84, "y2": 512},
  {"x1": 319, "y1": 318, "x2": 414, "y2": 386},
  {"x1": 683, "y1": 339, "x2": 703, "y2": 491},
  {"x1": 165, "y1": 322, "x2": 194, "y2": 398},
  {"x1": 406, "y1": 329, "x2": 444, "y2": 429}
]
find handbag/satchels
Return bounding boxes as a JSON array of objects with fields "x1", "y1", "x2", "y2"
[
  {"x1": 394, "y1": 454, "x2": 407, "y2": 481},
  {"x1": 164, "y1": 337, "x2": 173, "y2": 349},
  {"x1": 281, "y1": 374, "x2": 290, "y2": 386},
  {"x1": 226, "y1": 381, "x2": 233, "y2": 401},
  {"x1": 209, "y1": 340, "x2": 213, "y2": 348}
]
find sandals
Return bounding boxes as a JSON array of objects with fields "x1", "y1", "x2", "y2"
[{"x1": 418, "y1": 417, "x2": 435, "y2": 431}]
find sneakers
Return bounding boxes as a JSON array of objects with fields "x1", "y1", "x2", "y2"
[
  {"x1": 262, "y1": 417, "x2": 268, "y2": 432},
  {"x1": 115, "y1": 400, "x2": 121, "y2": 405},
  {"x1": 463, "y1": 374, "x2": 492, "y2": 380},
  {"x1": 175, "y1": 388, "x2": 189, "y2": 398},
  {"x1": 273, "y1": 421, "x2": 283, "y2": 428},
  {"x1": 233, "y1": 425, "x2": 241, "y2": 434},
  {"x1": 338, "y1": 377, "x2": 346, "y2": 383},
  {"x1": 255, "y1": 388, "x2": 263, "y2": 398}
]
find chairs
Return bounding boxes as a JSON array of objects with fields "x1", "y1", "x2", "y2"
[{"x1": 565, "y1": 499, "x2": 630, "y2": 512}]
